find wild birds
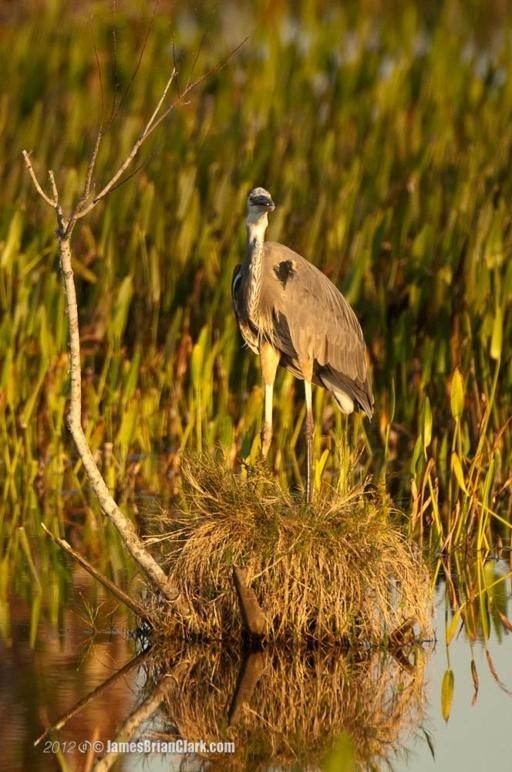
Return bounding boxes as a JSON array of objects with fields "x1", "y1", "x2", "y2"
[{"x1": 230, "y1": 185, "x2": 376, "y2": 503}]
